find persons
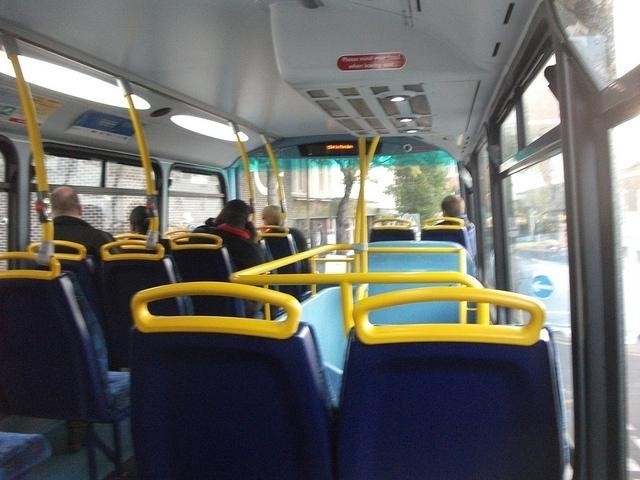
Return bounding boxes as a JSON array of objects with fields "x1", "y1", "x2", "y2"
[
  {"x1": 52, "y1": 184, "x2": 123, "y2": 259},
  {"x1": 127, "y1": 205, "x2": 168, "y2": 255},
  {"x1": 262, "y1": 204, "x2": 311, "y2": 291},
  {"x1": 190, "y1": 199, "x2": 279, "y2": 320},
  {"x1": 440, "y1": 194, "x2": 478, "y2": 258}
]
